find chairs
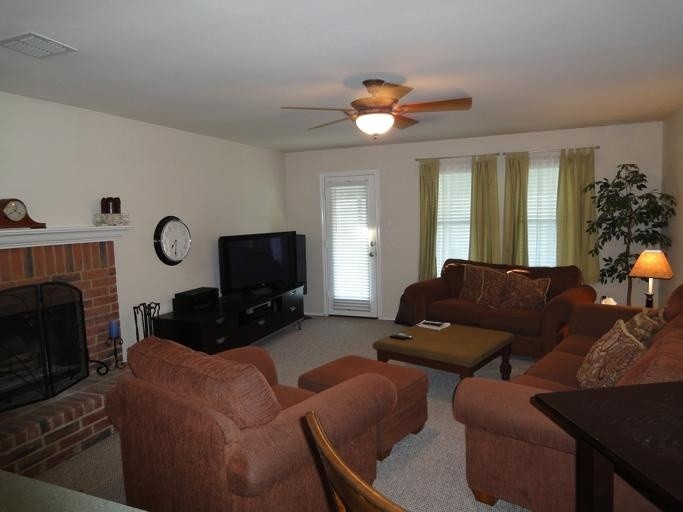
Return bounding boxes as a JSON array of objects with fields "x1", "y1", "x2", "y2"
[{"x1": 300, "y1": 411, "x2": 408, "y2": 511}]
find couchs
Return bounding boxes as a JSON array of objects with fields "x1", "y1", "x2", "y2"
[
  {"x1": 454, "y1": 285, "x2": 683, "y2": 512},
  {"x1": 102, "y1": 335, "x2": 397, "y2": 511},
  {"x1": 390, "y1": 258, "x2": 596, "y2": 355}
]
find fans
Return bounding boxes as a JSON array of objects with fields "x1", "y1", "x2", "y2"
[{"x1": 278, "y1": 78, "x2": 475, "y2": 132}]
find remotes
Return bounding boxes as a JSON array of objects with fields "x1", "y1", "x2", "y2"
[{"x1": 390, "y1": 333, "x2": 413, "y2": 340}]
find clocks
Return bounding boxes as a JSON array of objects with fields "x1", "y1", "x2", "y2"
[
  {"x1": 0, "y1": 198, "x2": 50, "y2": 229},
  {"x1": 156, "y1": 215, "x2": 193, "y2": 265}
]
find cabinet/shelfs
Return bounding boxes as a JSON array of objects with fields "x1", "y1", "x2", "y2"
[{"x1": 155, "y1": 285, "x2": 308, "y2": 355}]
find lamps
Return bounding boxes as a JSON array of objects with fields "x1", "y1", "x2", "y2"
[
  {"x1": 356, "y1": 113, "x2": 393, "y2": 139},
  {"x1": 629, "y1": 250, "x2": 673, "y2": 309}
]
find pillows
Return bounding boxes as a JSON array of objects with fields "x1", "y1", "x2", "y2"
[
  {"x1": 622, "y1": 311, "x2": 656, "y2": 348},
  {"x1": 499, "y1": 272, "x2": 552, "y2": 310},
  {"x1": 459, "y1": 264, "x2": 510, "y2": 303},
  {"x1": 643, "y1": 310, "x2": 666, "y2": 328},
  {"x1": 573, "y1": 318, "x2": 648, "y2": 391}
]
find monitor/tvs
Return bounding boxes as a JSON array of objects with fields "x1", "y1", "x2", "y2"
[{"x1": 219, "y1": 231, "x2": 298, "y2": 295}]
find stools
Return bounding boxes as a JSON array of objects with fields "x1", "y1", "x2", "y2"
[{"x1": 305, "y1": 354, "x2": 429, "y2": 460}]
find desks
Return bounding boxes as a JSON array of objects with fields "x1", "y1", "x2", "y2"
[{"x1": 526, "y1": 381, "x2": 683, "y2": 511}]
